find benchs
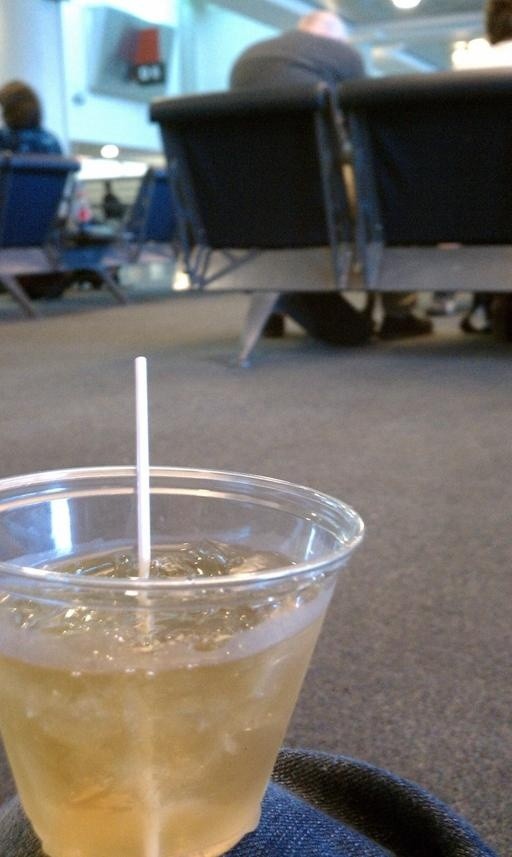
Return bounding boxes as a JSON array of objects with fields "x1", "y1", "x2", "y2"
[
  {"x1": 0, "y1": 151, "x2": 178, "y2": 317},
  {"x1": 151, "y1": 65, "x2": 511, "y2": 373}
]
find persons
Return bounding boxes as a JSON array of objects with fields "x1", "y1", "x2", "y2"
[
  {"x1": 229, "y1": 11, "x2": 432, "y2": 337},
  {"x1": 459, "y1": 0, "x2": 512, "y2": 332},
  {"x1": 0, "y1": 80, "x2": 61, "y2": 155}
]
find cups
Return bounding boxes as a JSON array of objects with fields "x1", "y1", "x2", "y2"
[{"x1": 0, "y1": 466, "x2": 368, "y2": 856}]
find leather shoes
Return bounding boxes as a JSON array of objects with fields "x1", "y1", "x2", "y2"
[{"x1": 379, "y1": 313, "x2": 434, "y2": 342}]
